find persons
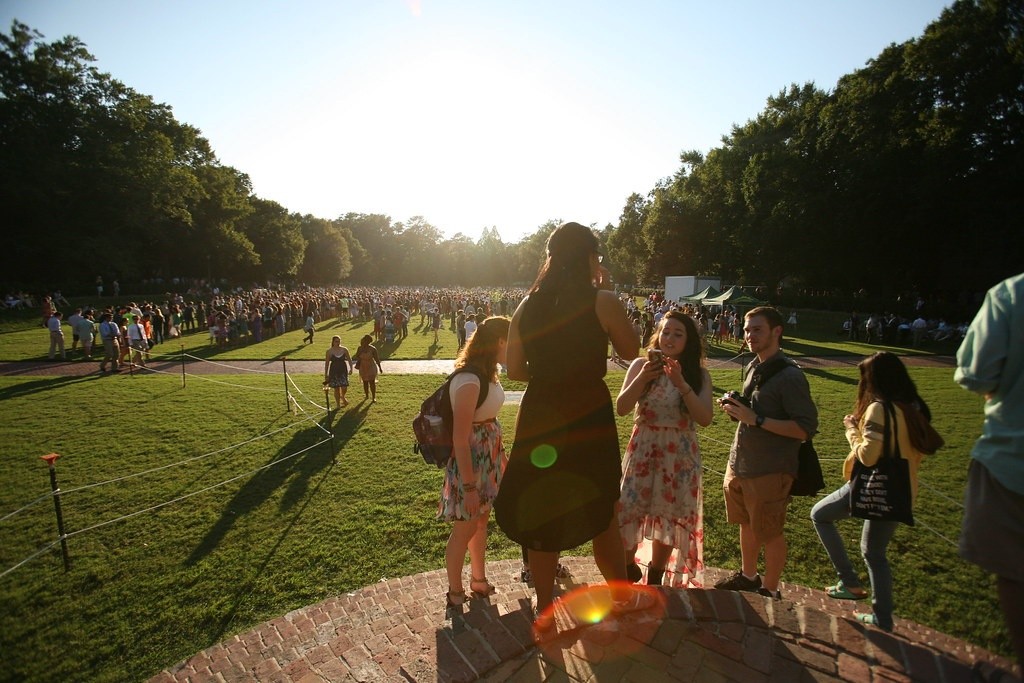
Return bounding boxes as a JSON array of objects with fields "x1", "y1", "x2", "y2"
[
  {"x1": 810, "y1": 351, "x2": 945, "y2": 627},
  {"x1": 41, "y1": 292, "x2": 195, "y2": 373},
  {"x1": 187, "y1": 285, "x2": 529, "y2": 346},
  {"x1": 617, "y1": 311, "x2": 713, "y2": 589},
  {"x1": 787, "y1": 309, "x2": 797, "y2": 328},
  {"x1": 843, "y1": 289, "x2": 970, "y2": 347},
  {"x1": 610, "y1": 289, "x2": 740, "y2": 362},
  {"x1": 493, "y1": 222, "x2": 656, "y2": 632},
  {"x1": 325, "y1": 335, "x2": 353, "y2": 407},
  {"x1": 0, "y1": 289, "x2": 70, "y2": 310},
  {"x1": 354, "y1": 335, "x2": 383, "y2": 402},
  {"x1": 755, "y1": 282, "x2": 768, "y2": 301},
  {"x1": 713, "y1": 307, "x2": 818, "y2": 599},
  {"x1": 434, "y1": 317, "x2": 511, "y2": 605},
  {"x1": 112, "y1": 277, "x2": 120, "y2": 296},
  {"x1": 96, "y1": 275, "x2": 104, "y2": 297},
  {"x1": 953, "y1": 274, "x2": 1024, "y2": 666}
]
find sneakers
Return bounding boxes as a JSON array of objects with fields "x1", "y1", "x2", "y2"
[
  {"x1": 757, "y1": 588, "x2": 783, "y2": 601},
  {"x1": 712, "y1": 569, "x2": 762, "y2": 592}
]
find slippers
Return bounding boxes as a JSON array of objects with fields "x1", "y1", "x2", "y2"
[
  {"x1": 824, "y1": 580, "x2": 868, "y2": 601},
  {"x1": 849, "y1": 611, "x2": 877, "y2": 626}
]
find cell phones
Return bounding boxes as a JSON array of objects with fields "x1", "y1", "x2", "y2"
[{"x1": 648, "y1": 350, "x2": 664, "y2": 371}]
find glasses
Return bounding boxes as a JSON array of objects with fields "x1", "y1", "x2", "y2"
[{"x1": 588, "y1": 247, "x2": 604, "y2": 265}]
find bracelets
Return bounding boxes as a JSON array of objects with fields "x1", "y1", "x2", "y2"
[
  {"x1": 463, "y1": 481, "x2": 477, "y2": 492},
  {"x1": 680, "y1": 389, "x2": 691, "y2": 398},
  {"x1": 501, "y1": 448, "x2": 505, "y2": 452}
]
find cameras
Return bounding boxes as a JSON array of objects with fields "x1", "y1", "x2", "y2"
[{"x1": 721, "y1": 391, "x2": 752, "y2": 423}]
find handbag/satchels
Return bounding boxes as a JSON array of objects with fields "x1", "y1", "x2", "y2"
[
  {"x1": 354, "y1": 360, "x2": 361, "y2": 369},
  {"x1": 789, "y1": 438, "x2": 825, "y2": 498},
  {"x1": 712, "y1": 322, "x2": 719, "y2": 329},
  {"x1": 302, "y1": 324, "x2": 310, "y2": 332},
  {"x1": 846, "y1": 398, "x2": 917, "y2": 530}
]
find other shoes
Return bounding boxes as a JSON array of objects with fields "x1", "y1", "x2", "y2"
[
  {"x1": 343, "y1": 399, "x2": 348, "y2": 405},
  {"x1": 556, "y1": 565, "x2": 575, "y2": 583},
  {"x1": 521, "y1": 569, "x2": 529, "y2": 582},
  {"x1": 303, "y1": 338, "x2": 307, "y2": 345},
  {"x1": 647, "y1": 562, "x2": 666, "y2": 586},
  {"x1": 626, "y1": 561, "x2": 643, "y2": 585},
  {"x1": 372, "y1": 398, "x2": 377, "y2": 402},
  {"x1": 334, "y1": 407, "x2": 342, "y2": 412}
]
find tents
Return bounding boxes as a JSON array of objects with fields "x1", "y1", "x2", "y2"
[{"x1": 678, "y1": 286, "x2": 770, "y2": 314}]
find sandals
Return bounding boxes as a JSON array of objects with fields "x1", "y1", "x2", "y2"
[
  {"x1": 469, "y1": 576, "x2": 502, "y2": 597},
  {"x1": 529, "y1": 593, "x2": 556, "y2": 631},
  {"x1": 610, "y1": 586, "x2": 658, "y2": 616},
  {"x1": 446, "y1": 585, "x2": 475, "y2": 608}
]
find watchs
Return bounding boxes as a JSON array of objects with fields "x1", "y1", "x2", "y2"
[{"x1": 755, "y1": 415, "x2": 765, "y2": 428}]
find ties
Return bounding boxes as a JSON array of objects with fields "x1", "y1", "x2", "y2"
[
  {"x1": 106, "y1": 324, "x2": 114, "y2": 335},
  {"x1": 135, "y1": 324, "x2": 144, "y2": 340}
]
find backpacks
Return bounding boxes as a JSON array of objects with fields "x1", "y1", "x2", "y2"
[{"x1": 414, "y1": 366, "x2": 489, "y2": 469}]
